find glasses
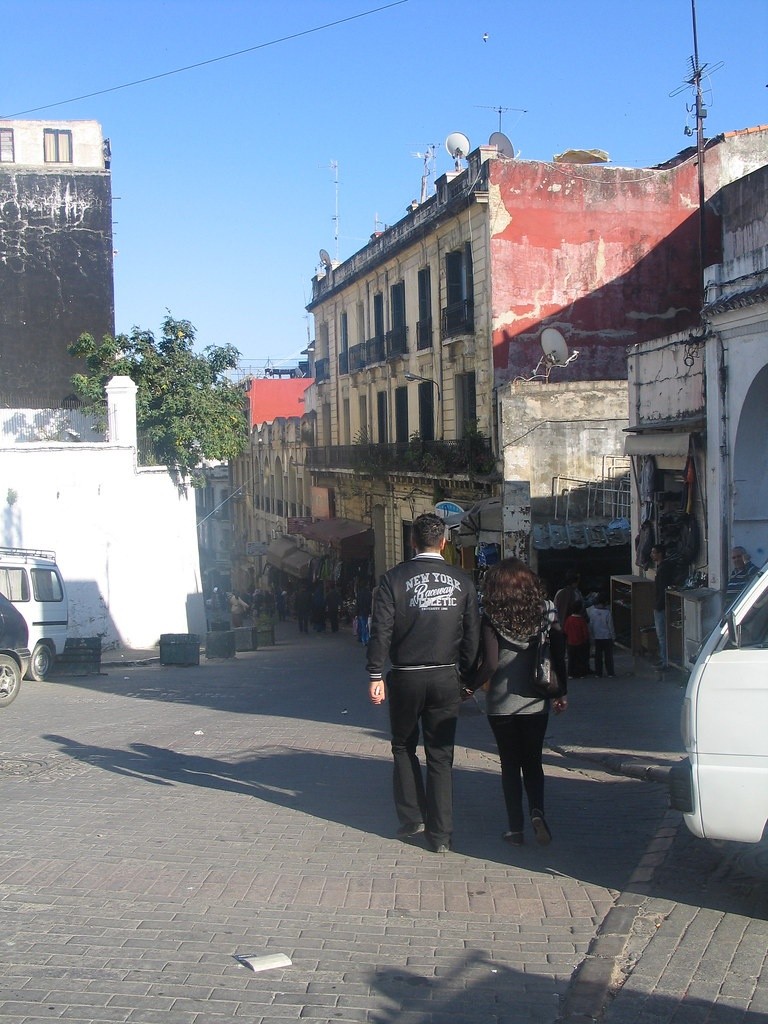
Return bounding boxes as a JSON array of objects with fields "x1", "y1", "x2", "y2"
[{"x1": 732, "y1": 555, "x2": 741, "y2": 559}]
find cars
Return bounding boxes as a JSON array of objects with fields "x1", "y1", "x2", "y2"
[{"x1": 0, "y1": 593, "x2": 31, "y2": 707}]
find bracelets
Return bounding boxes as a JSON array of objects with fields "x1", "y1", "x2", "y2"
[{"x1": 744, "y1": 560, "x2": 750, "y2": 564}]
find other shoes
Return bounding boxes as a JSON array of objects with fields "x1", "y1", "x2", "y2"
[
  {"x1": 568, "y1": 675, "x2": 575, "y2": 679},
  {"x1": 397, "y1": 821, "x2": 425, "y2": 836},
  {"x1": 579, "y1": 675, "x2": 585, "y2": 679},
  {"x1": 652, "y1": 661, "x2": 663, "y2": 668},
  {"x1": 654, "y1": 667, "x2": 668, "y2": 672},
  {"x1": 433, "y1": 840, "x2": 451, "y2": 853},
  {"x1": 594, "y1": 675, "x2": 601, "y2": 678},
  {"x1": 531, "y1": 810, "x2": 552, "y2": 846},
  {"x1": 608, "y1": 674, "x2": 617, "y2": 678},
  {"x1": 503, "y1": 831, "x2": 524, "y2": 847}
]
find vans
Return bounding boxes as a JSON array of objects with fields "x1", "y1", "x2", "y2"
[
  {"x1": 664, "y1": 555, "x2": 768, "y2": 848},
  {"x1": 0, "y1": 546, "x2": 69, "y2": 682}
]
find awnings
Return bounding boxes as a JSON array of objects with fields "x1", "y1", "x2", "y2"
[
  {"x1": 623, "y1": 432, "x2": 709, "y2": 530},
  {"x1": 266, "y1": 516, "x2": 374, "y2": 579},
  {"x1": 532, "y1": 522, "x2": 632, "y2": 550}
]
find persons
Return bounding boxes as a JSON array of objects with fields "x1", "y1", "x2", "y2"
[
  {"x1": 554, "y1": 571, "x2": 590, "y2": 674},
  {"x1": 366, "y1": 513, "x2": 478, "y2": 851},
  {"x1": 213, "y1": 583, "x2": 369, "y2": 643},
  {"x1": 650, "y1": 544, "x2": 675, "y2": 670},
  {"x1": 590, "y1": 596, "x2": 617, "y2": 678},
  {"x1": 565, "y1": 600, "x2": 590, "y2": 679},
  {"x1": 724, "y1": 547, "x2": 761, "y2": 615},
  {"x1": 461, "y1": 558, "x2": 568, "y2": 844}
]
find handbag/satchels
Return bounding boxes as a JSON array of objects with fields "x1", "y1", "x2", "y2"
[{"x1": 535, "y1": 600, "x2": 568, "y2": 698}]
